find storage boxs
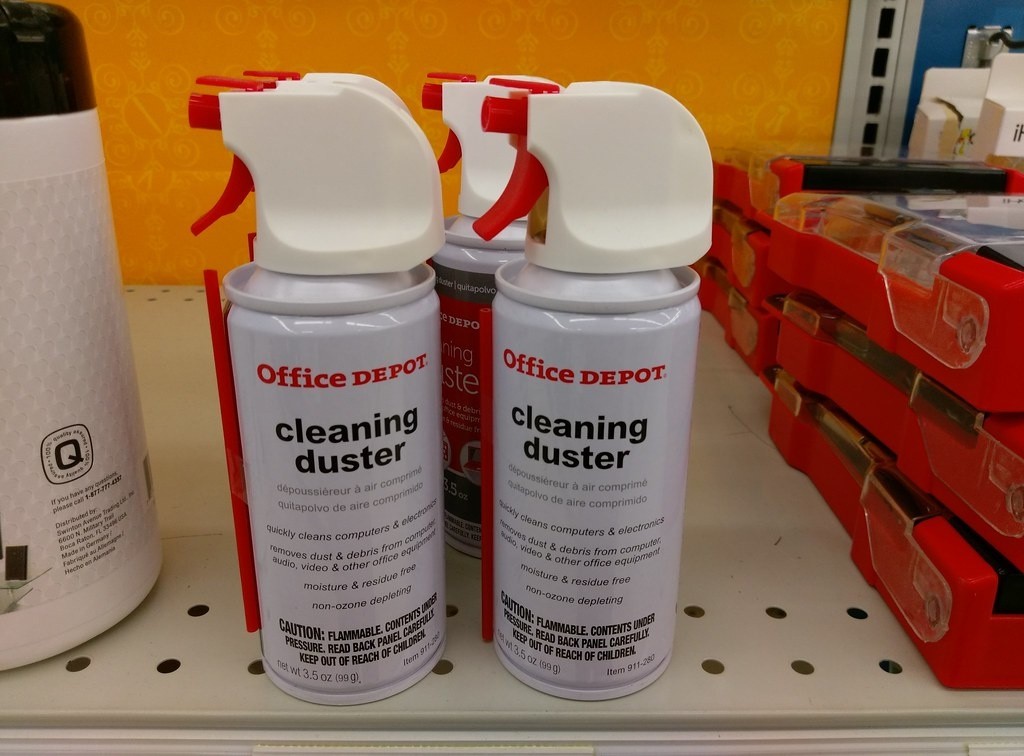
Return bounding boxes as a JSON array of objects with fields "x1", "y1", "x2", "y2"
[{"x1": 694, "y1": 46, "x2": 1024, "y2": 692}]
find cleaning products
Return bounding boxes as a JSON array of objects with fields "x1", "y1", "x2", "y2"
[{"x1": 187, "y1": 71, "x2": 715, "y2": 708}]
